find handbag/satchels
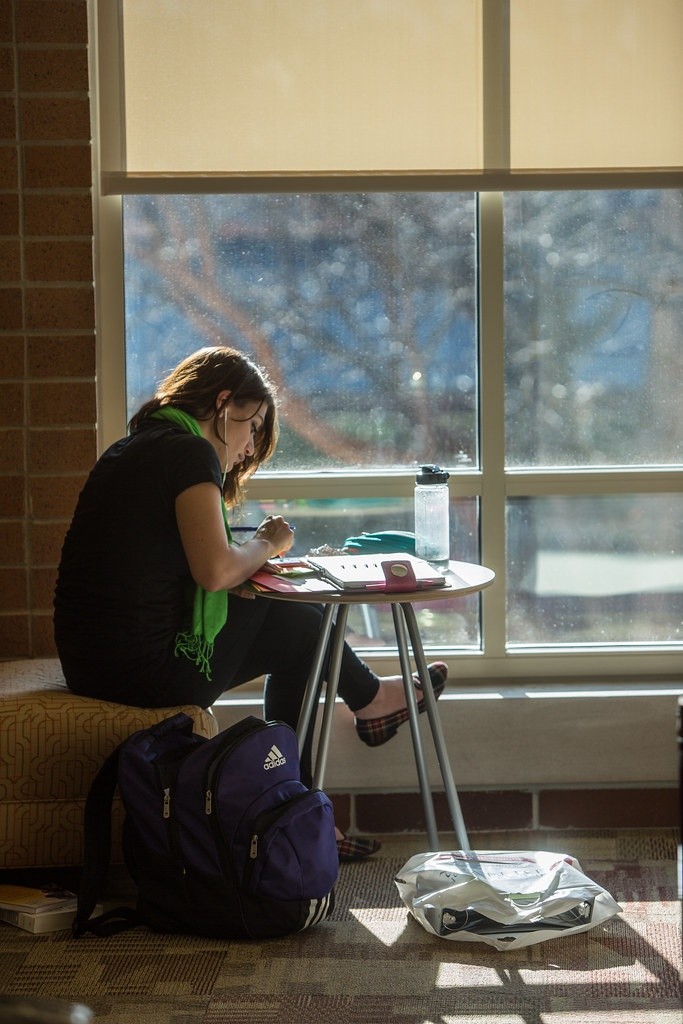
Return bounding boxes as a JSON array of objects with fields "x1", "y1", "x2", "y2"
[{"x1": 393, "y1": 850, "x2": 623, "y2": 952}]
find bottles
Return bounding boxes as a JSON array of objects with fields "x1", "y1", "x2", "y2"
[{"x1": 415, "y1": 463, "x2": 451, "y2": 569}]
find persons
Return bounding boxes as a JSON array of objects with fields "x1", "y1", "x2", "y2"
[{"x1": 53, "y1": 346, "x2": 447, "y2": 865}]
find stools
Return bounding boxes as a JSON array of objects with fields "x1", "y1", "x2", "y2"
[{"x1": 0, "y1": 658, "x2": 218, "y2": 866}]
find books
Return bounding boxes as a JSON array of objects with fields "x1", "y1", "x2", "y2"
[
  {"x1": 0, "y1": 885, "x2": 102, "y2": 934},
  {"x1": 298, "y1": 551, "x2": 452, "y2": 593}
]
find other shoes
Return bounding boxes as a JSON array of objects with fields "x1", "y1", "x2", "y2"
[
  {"x1": 335, "y1": 831, "x2": 382, "y2": 861},
  {"x1": 354, "y1": 660, "x2": 448, "y2": 747}
]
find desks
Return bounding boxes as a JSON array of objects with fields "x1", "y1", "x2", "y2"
[{"x1": 233, "y1": 557, "x2": 496, "y2": 851}]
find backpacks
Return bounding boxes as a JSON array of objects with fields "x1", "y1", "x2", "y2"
[{"x1": 71, "y1": 711, "x2": 339, "y2": 939}]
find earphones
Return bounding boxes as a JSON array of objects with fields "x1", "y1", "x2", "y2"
[{"x1": 223, "y1": 407, "x2": 229, "y2": 422}]
